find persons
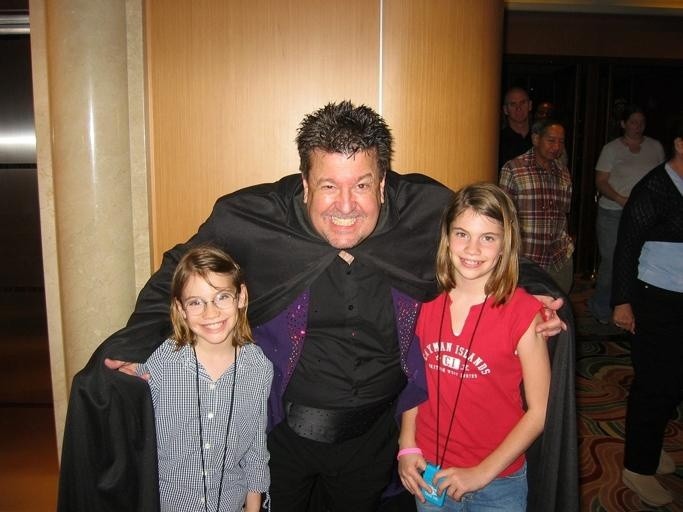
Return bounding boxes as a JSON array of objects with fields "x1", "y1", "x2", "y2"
[
  {"x1": 386, "y1": 179, "x2": 553, "y2": 510},
  {"x1": 606, "y1": 113, "x2": 683, "y2": 508},
  {"x1": 592, "y1": 102, "x2": 665, "y2": 327},
  {"x1": 496, "y1": 116, "x2": 576, "y2": 294},
  {"x1": 497, "y1": 86, "x2": 535, "y2": 166},
  {"x1": 129, "y1": 245, "x2": 275, "y2": 511},
  {"x1": 102, "y1": 100, "x2": 569, "y2": 511}
]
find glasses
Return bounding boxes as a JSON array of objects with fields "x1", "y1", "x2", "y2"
[{"x1": 182, "y1": 292, "x2": 238, "y2": 315}]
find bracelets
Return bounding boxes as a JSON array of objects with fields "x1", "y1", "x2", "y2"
[{"x1": 395, "y1": 447, "x2": 422, "y2": 461}]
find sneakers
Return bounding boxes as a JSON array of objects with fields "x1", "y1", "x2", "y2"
[
  {"x1": 657, "y1": 450, "x2": 674, "y2": 475},
  {"x1": 622, "y1": 469, "x2": 673, "y2": 507}
]
